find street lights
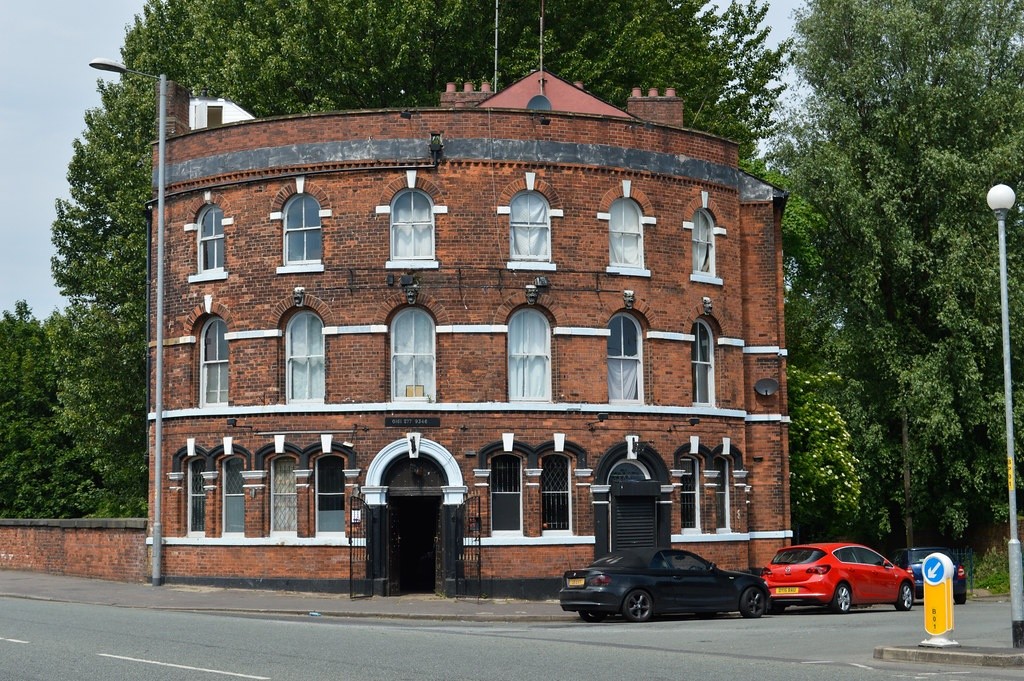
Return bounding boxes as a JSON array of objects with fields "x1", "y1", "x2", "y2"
[
  {"x1": 90, "y1": 58, "x2": 164, "y2": 585},
  {"x1": 986, "y1": 183, "x2": 1024, "y2": 649}
]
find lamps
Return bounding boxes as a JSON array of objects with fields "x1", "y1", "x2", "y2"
[
  {"x1": 226, "y1": 418, "x2": 253, "y2": 431},
  {"x1": 587, "y1": 413, "x2": 608, "y2": 425},
  {"x1": 429, "y1": 132, "x2": 444, "y2": 167}
]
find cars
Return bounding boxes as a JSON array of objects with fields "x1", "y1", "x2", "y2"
[
  {"x1": 895, "y1": 547, "x2": 967, "y2": 605},
  {"x1": 557, "y1": 547, "x2": 770, "y2": 623},
  {"x1": 760, "y1": 543, "x2": 916, "y2": 613}
]
